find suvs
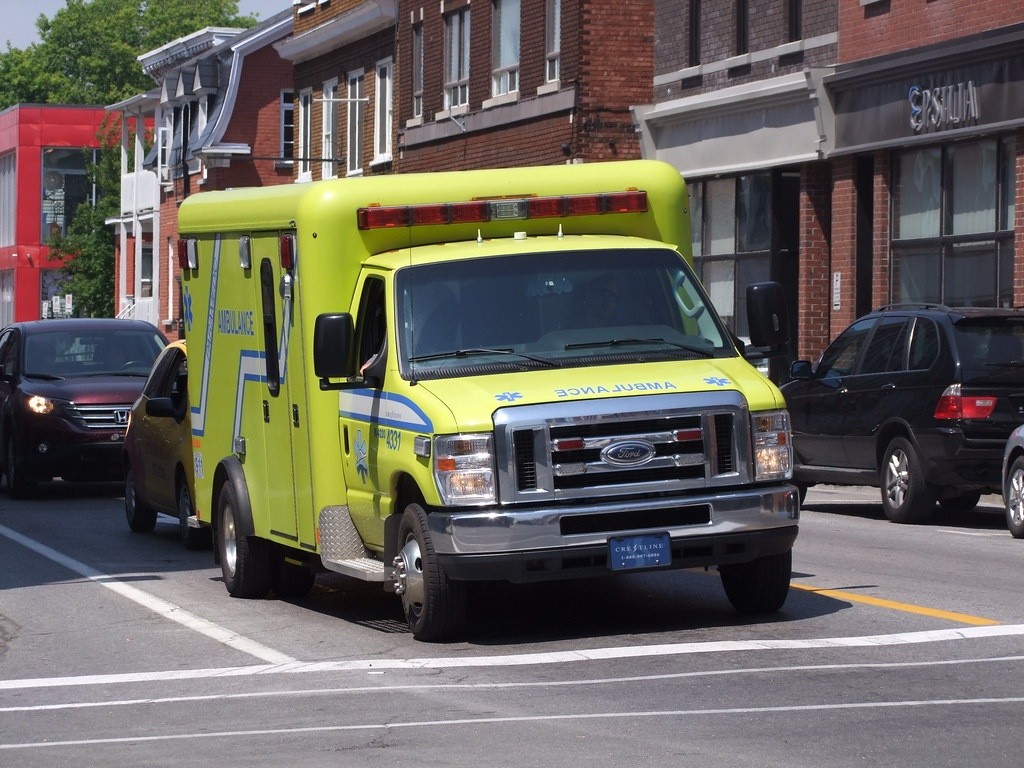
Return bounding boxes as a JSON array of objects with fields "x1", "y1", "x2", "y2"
[{"x1": 778, "y1": 303, "x2": 1023, "y2": 521}]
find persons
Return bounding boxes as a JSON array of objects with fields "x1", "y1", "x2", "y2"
[
  {"x1": 567, "y1": 276, "x2": 628, "y2": 330},
  {"x1": 359, "y1": 353, "x2": 382, "y2": 378}
]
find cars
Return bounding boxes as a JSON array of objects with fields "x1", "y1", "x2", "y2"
[
  {"x1": 119, "y1": 338, "x2": 213, "y2": 548},
  {"x1": 1, "y1": 316, "x2": 171, "y2": 499}
]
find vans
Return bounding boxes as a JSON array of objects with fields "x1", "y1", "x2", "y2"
[{"x1": 167, "y1": 158, "x2": 801, "y2": 639}]
